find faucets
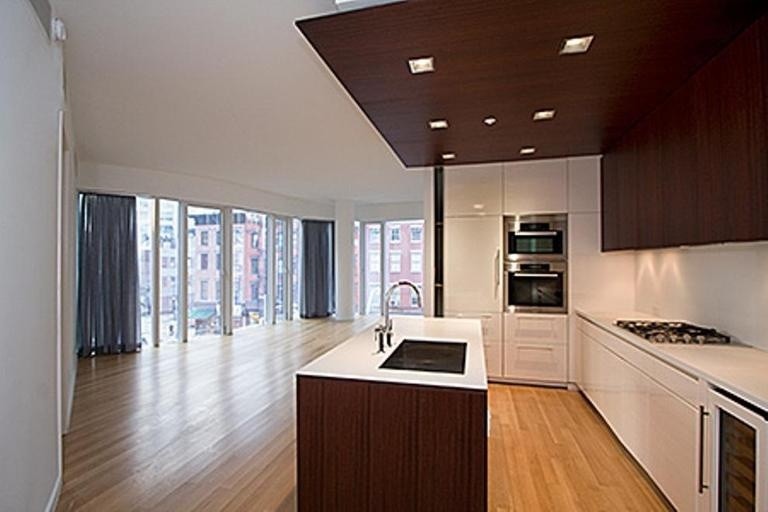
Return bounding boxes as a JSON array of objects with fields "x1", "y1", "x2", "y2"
[{"x1": 373, "y1": 280, "x2": 421, "y2": 351}]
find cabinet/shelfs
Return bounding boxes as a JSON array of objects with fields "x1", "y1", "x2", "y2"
[
  {"x1": 445, "y1": 313, "x2": 570, "y2": 388},
  {"x1": 444, "y1": 153, "x2": 568, "y2": 216},
  {"x1": 443, "y1": 215, "x2": 505, "y2": 312},
  {"x1": 698, "y1": 382, "x2": 768, "y2": 511},
  {"x1": 574, "y1": 314, "x2": 699, "y2": 512}
]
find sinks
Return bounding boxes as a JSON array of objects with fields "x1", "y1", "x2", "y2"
[{"x1": 378, "y1": 337, "x2": 467, "y2": 374}]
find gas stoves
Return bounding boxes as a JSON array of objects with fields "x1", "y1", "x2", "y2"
[{"x1": 613, "y1": 318, "x2": 754, "y2": 349}]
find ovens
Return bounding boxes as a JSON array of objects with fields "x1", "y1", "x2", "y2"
[{"x1": 503, "y1": 214, "x2": 567, "y2": 314}]
